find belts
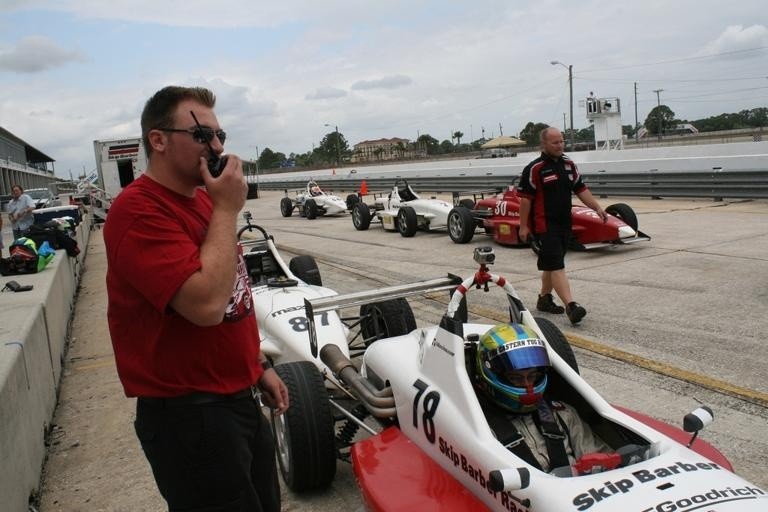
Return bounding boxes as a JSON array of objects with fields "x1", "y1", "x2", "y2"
[{"x1": 140, "y1": 384, "x2": 259, "y2": 409}]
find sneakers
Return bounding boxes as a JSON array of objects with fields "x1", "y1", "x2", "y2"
[
  {"x1": 537, "y1": 293, "x2": 564, "y2": 314},
  {"x1": 566, "y1": 302, "x2": 586, "y2": 324}
]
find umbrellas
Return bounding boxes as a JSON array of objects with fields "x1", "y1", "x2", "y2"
[{"x1": 479, "y1": 136, "x2": 527, "y2": 154}]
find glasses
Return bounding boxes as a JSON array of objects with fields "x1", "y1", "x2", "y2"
[{"x1": 157, "y1": 126, "x2": 226, "y2": 145}]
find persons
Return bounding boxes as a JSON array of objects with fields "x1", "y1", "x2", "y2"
[
  {"x1": 515, "y1": 125, "x2": 608, "y2": 324},
  {"x1": 470, "y1": 319, "x2": 644, "y2": 481},
  {"x1": 100, "y1": 85, "x2": 290, "y2": 512},
  {"x1": 587, "y1": 91, "x2": 597, "y2": 112},
  {"x1": 7, "y1": 184, "x2": 36, "y2": 240}
]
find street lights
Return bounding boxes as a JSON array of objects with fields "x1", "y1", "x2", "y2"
[
  {"x1": 324, "y1": 124, "x2": 342, "y2": 168},
  {"x1": 550, "y1": 61, "x2": 575, "y2": 152}
]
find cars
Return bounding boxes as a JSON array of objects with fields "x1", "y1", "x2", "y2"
[
  {"x1": 24, "y1": 187, "x2": 54, "y2": 208},
  {"x1": 475, "y1": 148, "x2": 515, "y2": 160}
]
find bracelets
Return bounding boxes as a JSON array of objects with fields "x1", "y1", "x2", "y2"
[
  {"x1": 261, "y1": 360, "x2": 272, "y2": 370},
  {"x1": 24, "y1": 208, "x2": 27, "y2": 213}
]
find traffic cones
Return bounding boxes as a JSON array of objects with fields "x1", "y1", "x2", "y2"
[{"x1": 332, "y1": 170, "x2": 337, "y2": 176}]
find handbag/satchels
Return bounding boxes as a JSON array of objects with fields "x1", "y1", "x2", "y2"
[
  {"x1": 30, "y1": 221, "x2": 62, "y2": 236},
  {"x1": 0, "y1": 254, "x2": 39, "y2": 276}
]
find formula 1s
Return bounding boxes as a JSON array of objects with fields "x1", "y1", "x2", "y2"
[
  {"x1": 446, "y1": 177, "x2": 652, "y2": 257},
  {"x1": 351, "y1": 178, "x2": 455, "y2": 237},
  {"x1": 236, "y1": 210, "x2": 420, "y2": 497},
  {"x1": 279, "y1": 179, "x2": 349, "y2": 220},
  {"x1": 265, "y1": 242, "x2": 768, "y2": 512}
]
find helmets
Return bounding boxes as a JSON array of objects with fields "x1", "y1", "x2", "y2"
[
  {"x1": 475, "y1": 322, "x2": 551, "y2": 414},
  {"x1": 8, "y1": 237, "x2": 39, "y2": 256}
]
